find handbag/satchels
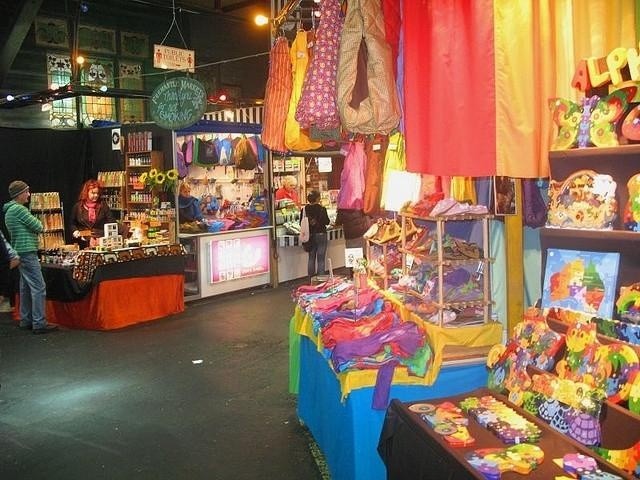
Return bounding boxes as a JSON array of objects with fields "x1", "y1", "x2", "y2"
[{"x1": 301, "y1": 207, "x2": 325, "y2": 252}]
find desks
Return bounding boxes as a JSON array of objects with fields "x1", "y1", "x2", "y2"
[
  {"x1": 180, "y1": 226, "x2": 275, "y2": 298},
  {"x1": 289, "y1": 272, "x2": 508, "y2": 480},
  {"x1": 377, "y1": 386, "x2": 640, "y2": 480},
  {"x1": 16, "y1": 254, "x2": 185, "y2": 331}
]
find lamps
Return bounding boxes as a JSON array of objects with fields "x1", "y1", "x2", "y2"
[{"x1": 255, "y1": 14, "x2": 270, "y2": 26}]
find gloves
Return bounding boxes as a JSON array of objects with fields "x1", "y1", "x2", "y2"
[{"x1": 72, "y1": 230, "x2": 81, "y2": 238}]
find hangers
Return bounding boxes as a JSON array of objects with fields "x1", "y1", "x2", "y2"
[
  {"x1": 276, "y1": 19, "x2": 288, "y2": 39},
  {"x1": 308, "y1": 7, "x2": 318, "y2": 32},
  {"x1": 297, "y1": 8, "x2": 306, "y2": 35}
]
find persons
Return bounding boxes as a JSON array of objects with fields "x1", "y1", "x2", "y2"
[
  {"x1": 3, "y1": 181, "x2": 59, "y2": 335},
  {"x1": 0, "y1": 226, "x2": 21, "y2": 270},
  {"x1": 177, "y1": 182, "x2": 204, "y2": 225},
  {"x1": 71, "y1": 179, "x2": 117, "y2": 250},
  {"x1": 276, "y1": 173, "x2": 299, "y2": 206},
  {"x1": 300, "y1": 190, "x2": 331, "y2": 283}
]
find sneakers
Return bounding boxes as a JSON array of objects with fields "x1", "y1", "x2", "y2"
[
  {"x1": 32, "y1": 325, "x2": 58, "y2": 335},
  {"x1": 19, "y1": 325, "x2": 32, "y2": 330}
]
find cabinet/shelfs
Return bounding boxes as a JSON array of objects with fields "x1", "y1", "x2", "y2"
[
  {"x1": 270, "y1": 152, "x2": 304, "y2": 221},
  {"x1": 275, "y1": 225, "x2": 347, "y2": 283},
  {"x1": 123, "y1": 130, "x2": 177, "y2": 246},
  {"x1": 97, "y1": 171, "x2": 126, "y2": 236},
  {"x1": 536, "y1": 141, "x2": 640, "y2": 478},
  {"x1": 363, "y1": 208, "x2": 496, "y2": 325},
  {"x1": 30, "y1": 193, "x2": 67, "y2": 252}
]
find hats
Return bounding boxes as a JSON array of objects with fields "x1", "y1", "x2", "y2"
[{"x1": 8, "y1": 180, "x2": 30, "y2": 199}]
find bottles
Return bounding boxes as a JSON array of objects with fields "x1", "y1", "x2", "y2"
[
  {"x1": 38, "y1": 233, "x2": 65, "y2": 250},
  {"x1": 30, "y1": 191, "x2": 60, "y2": 209},
  {"x1": 130, "y1": 206, "x2": 148, "y2": 219},
  {"x1": 127, "y1": 131, "x2": 152, "y2": 151},
  {"x1": 33, "y1": 212, "x2": 63, "y2": 230},
  {"x1": 127, "y1": 153, "x2": 151, "y2": 166},
  {"x1": 128, "y1": 172, "x2": 141, "y2": 183},
  {"x1": 101, "y1": 188, "x2": 118, "y2": 208},
  {"x1": 97, "y1": 171, "x2": 124, "y2": 186},
  {"x1": 131, "y1": 191, "x2": 152, "y2": 202}
]
